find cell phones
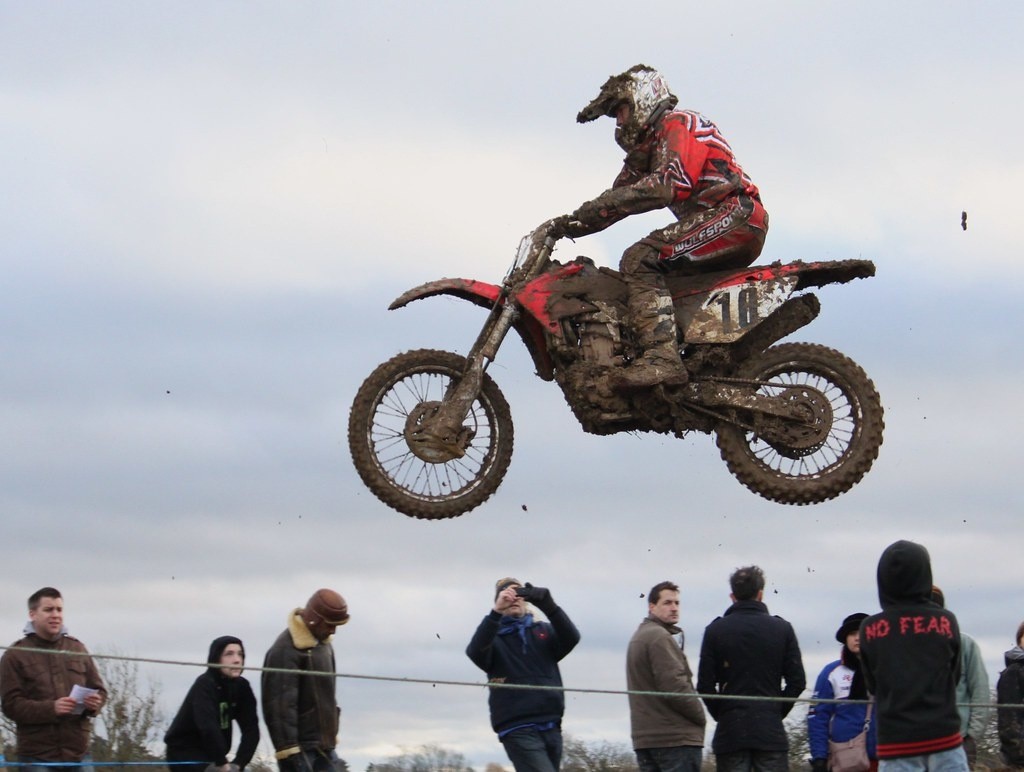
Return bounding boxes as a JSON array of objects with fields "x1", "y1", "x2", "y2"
[{"x1": 514, "y1": 588, "x2": 530, "y2": 596}]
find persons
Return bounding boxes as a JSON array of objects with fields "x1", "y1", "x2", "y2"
[
  {"x1": 696, "y1": 566, "x2": 806, "y2": 772},
  {"x1": 164, "y1": 636, "x2": 260, "y2": 772},
  {"x1": 997, "y1": 622, "x2": 1024, "y2": 772},
  {"x1": 260, "y1": 589, "x2": 350, "y2": 772},
  {"x1": 806, "y1": 540, "x2": 989, "y2": 772},
  {"x1": 466, "y1": 577, "x2": 580, "y2": 772},
  {"x1": 577, "y1": 63, "x2": 767, "y2": 391},
  {"x1": 0, "y1": 586, "x2": 107, "y2": 772},
  {"x1": 626, "y1": 581, "x2": 707, "y2": 772}
]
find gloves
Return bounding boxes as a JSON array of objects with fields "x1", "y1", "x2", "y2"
[
  {"x1": 575, "y1": 196, "x2": 622, "y2": 231},
  {"x1": 514, "y1": 582, "x2": 558, "y2": 617},
  {"x1": 809, "y1": 758, "x2": 826, "y2": 772},
  {"x1": 278, "y1": 756, "x2": 301, "y2": 772},
  {"x1": 532, "y1": 215, "x2": 572, "y2": 251}
]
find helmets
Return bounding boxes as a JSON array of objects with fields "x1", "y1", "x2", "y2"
[{"x1": 577, "y1": 64, "x2": 676, "y2": 153}]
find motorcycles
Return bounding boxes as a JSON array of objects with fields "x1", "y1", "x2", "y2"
[{"x1": 347, "y1": 219, "x2": 885, "y2": 521}]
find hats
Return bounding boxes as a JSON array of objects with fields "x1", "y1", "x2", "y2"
[
  {"x1": 310, "y1": 588, "x2": 350, "y2": 624},
  {"x1": 836, "y1": 613, "x2": 869, "y2": 643}
]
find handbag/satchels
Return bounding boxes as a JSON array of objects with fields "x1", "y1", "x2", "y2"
[{"x1": 826, "y1": 731, "x2": 871, "y2": 772}]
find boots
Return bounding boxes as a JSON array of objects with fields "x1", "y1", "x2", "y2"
[{"x1": 607, "y1": 290, "x2": 688, "y2": 392}]
